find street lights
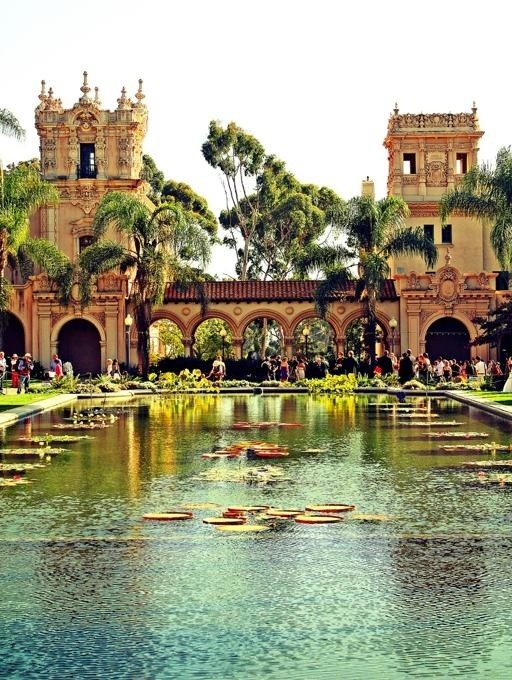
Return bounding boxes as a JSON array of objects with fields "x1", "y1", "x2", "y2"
[
  {"x1": 389, "y1": 316, "x2": 397, "y2": 353},
  {"x1": 125, "y1": 314, "x2": 133, "y2": 368},
  {"x1": 220, "y1": 327, "x2": 227, "y2": 363},
  {"x1": 303, "y1": 326, "x2": 310, "y2": 358}
]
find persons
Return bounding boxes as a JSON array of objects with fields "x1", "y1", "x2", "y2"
[
  {"x1": 55, "y1": 360, "x2": 62, "y2": 376},
  {"x1": 1, "y1": 351, "x2": 7, "y2": 389},
  {"x1": 212, "y1": 354, "x2": 227, "y2": 383},
  {"x1": 16, "y1": 353, "x2": 36, "y2": 394},
  {"x1": 106, "y1": 358, "x2": 113, "y2": 372},
  {"x1": 260, "y1": 348, "x2": 512, "y2": 384},
  {"x1": 11, "y1": 353, "x2": 20, "y2": 387},
  {"x1": 113, "y1": 358, "x2": 120, "y2": 372},
  {"x1": 51, "y1": 354, "x2": 63, "y2": 372}
]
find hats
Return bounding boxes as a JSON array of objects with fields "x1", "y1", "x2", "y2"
[
  {"x1": 25, "y1": 353, "x2": 31, "y2": 358},
  {"x1": 53, "y1": 355, "x2": 57, "y2": 359},
  {"x1": 12, "y1": 354, "x2": 18, "y2": 357},
  {"x1": 213, "y1": 360, "x2": 219, "y2": 366}
]
